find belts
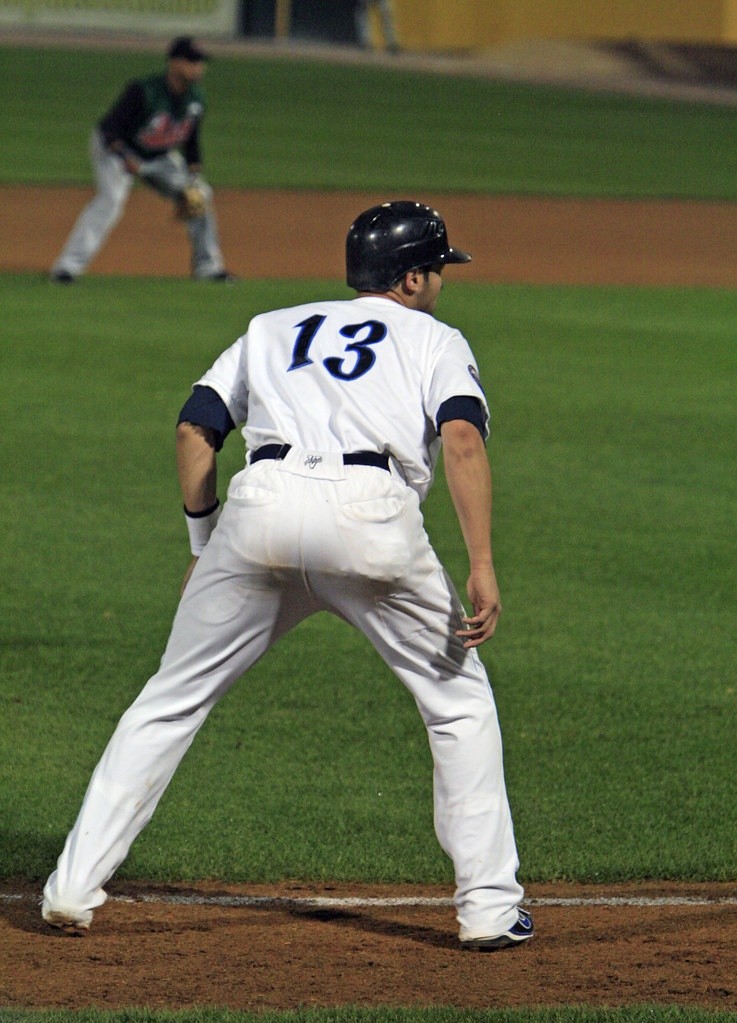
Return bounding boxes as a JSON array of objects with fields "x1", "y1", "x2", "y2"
[{"x1": 249, "y1": 444, "x2": 390, "y2": 472}]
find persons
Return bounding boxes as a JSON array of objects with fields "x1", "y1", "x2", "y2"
[
  {"x1": 40, "y1": 201, "x2": 538, "y2": 954},
  {"x1": 50, "y1": 35, "x2": 236, "y2": 285},
  {"x1": 353, "y1": 0, "x2": 411, "y2": 56}
]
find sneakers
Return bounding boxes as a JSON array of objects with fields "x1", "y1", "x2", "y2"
[
  {"x1": 462, "y1": 907, "x2": 536, "y2": 950},
  {"x1": 44, "y1": 909, "x2": 92, "y2": 937}
]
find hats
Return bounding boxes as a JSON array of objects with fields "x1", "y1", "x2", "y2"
[{"x1": 169, "y1": 34, "x2": 211, "y2": 60}]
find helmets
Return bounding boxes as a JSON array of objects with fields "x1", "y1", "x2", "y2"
[{"x1": 345, "y1": 201, "x2": 473, "y2": 294}]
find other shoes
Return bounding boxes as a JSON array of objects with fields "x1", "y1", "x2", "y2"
[
  {"x1": 209, "y1": 271, "x2": 235, "y2": 282},
  {"x1": 54, "y1": 270, "x2": 74, "y2": 284}
]
